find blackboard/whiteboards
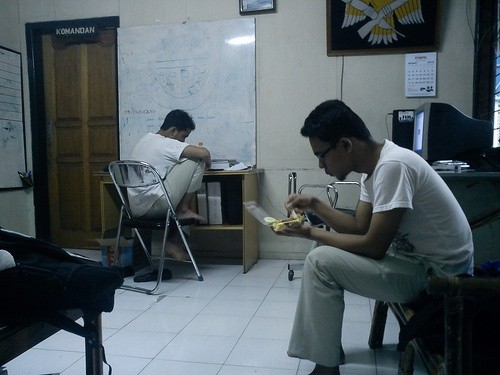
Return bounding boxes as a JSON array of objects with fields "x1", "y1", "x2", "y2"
[{"x1": 115, "y1": 17, "x2": 258, "y2": 167}]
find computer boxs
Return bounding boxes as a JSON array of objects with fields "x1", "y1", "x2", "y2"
[{"x1": 391, "y1": 109, "x2": 415, "y2": 150}]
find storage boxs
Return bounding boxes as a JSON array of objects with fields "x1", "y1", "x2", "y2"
[{"x1": 96, "y1": 232, "x2": 134, "y2": 267}]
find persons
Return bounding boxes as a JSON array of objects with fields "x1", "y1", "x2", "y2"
[
  {"x1": 271, "y1": 99, "x2": 474, "y2": 375},
  {"x1": 126, "y1": 109, "x2": 210, "y2": 261}
]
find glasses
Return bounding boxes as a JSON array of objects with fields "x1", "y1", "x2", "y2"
[{"x1": 316, "y1": 143, "x2": 336, "y2": 162}]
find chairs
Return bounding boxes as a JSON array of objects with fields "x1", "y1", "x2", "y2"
[
  {"x1": 288, "y1": 172, "x2": 361, "y2": 282},
  {"x1": 108, "y1": 160, "x2": 204, "y2": 295},
  {"x1": 367, "y1": 208, "x2": 500, "y2": 375},
  {"x1": 0, "y1": 227, "x2": 124, "y2": 375}
]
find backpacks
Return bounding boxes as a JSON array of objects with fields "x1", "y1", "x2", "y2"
[{"x1": 0, "y1": 227, "x2": 125, "y2": 375}]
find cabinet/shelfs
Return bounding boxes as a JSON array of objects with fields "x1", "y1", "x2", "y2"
[{"x1": 92, "y1": 168, "x2": 265, "y2": 274}]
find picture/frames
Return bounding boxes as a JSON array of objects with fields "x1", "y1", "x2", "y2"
[
  {"x1": 325, "y1": 0, "x2": 438, "y2": 57},
  {"x1": 238, "y1": 0, "x2": 276, "y2": 16}
]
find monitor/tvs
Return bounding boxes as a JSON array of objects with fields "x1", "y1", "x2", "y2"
[{"x1": 413, "y1": 102, "x2": 493, "y2": 163}]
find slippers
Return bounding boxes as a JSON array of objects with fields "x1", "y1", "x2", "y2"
[{"x1": 134, "y1": 269, "x2": 172, "y2": 282}]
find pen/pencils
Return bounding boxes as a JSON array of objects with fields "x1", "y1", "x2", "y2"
[{"x1": 18, "y1": 169, "x2": 33, "y2": 188}]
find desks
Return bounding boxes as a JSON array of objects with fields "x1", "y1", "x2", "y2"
[{"x1": 435, "y1": 170, "x2": 500, "y2": 269}]
